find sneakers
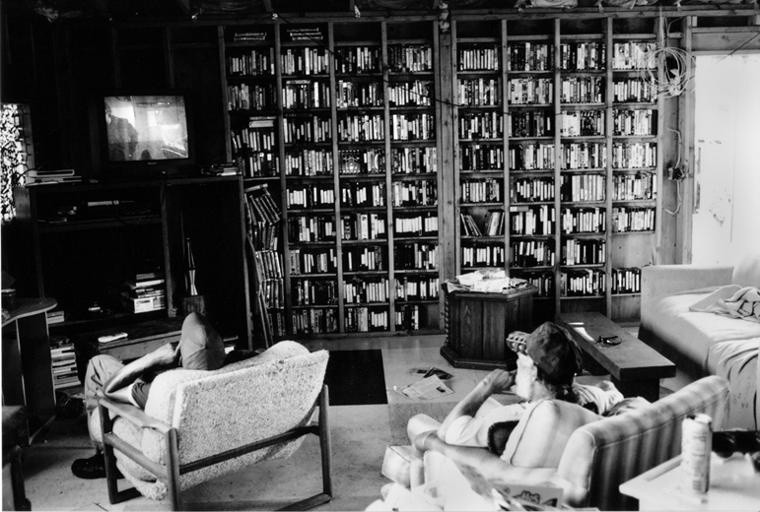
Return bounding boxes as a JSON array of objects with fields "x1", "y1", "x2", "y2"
[{"x1": 72, "y1": 448, "x2": 121, "y2": 478}]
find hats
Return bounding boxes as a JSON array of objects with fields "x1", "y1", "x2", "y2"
[
  {"x1": 180, "y1": 312, "x2": 225, "y2": 370},
  {"x1": 506, "y1": 321, "x2": 582, "y2": 386}
]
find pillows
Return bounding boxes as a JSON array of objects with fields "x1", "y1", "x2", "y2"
[{"x1": 500, "y1": 396, "x2": 606, "y2": 467}]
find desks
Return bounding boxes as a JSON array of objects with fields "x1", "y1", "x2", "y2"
[
  {"x1": 3, "y1": 297, "x2": 70, "y2": 446},
  {"x1": 439, "y1": 279, "x2": 540, "y2": 370}
]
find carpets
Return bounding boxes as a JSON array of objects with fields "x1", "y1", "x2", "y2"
[{"x1": 310, "y1": 349, "x2": 388, "y2": 405}]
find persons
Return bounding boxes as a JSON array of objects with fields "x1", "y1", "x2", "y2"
[
  {"x1": 71, "y1": 307, "x2": 267, "y2": 483},
  {"x1": 394, "y1": 319, "x2": 609, "y2": 492}
]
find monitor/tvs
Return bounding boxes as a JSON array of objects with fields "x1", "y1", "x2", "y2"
[{"x1": 95, "y1": 87, "x2": 192, "y2": 166}]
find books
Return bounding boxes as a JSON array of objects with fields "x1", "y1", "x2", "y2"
[{"x1": 44, "y1": 261, "x2": 167, "y2": 396}]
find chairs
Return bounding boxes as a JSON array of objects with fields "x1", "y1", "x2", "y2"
[
  {"x1": 96, "y1": 339, "x2": 335, "y2": 512},
  {"x1": 381, "y1": 371, "x2": 729, "y2": 509}
]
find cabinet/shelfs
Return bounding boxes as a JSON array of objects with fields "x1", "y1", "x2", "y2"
[
  {"x1": 210, "y1": 9, "x2": 442, "y2": 339},
  {"x1": 14, "y1": 172, "x2": 260, "y2": 422},
  {"x1": 444, "y1": 12, "x2": 664, "y2": 328}
]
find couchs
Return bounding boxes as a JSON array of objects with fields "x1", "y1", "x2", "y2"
[{"x1": 635, "y1": 255, "x2": 758, "y2": 379}]
